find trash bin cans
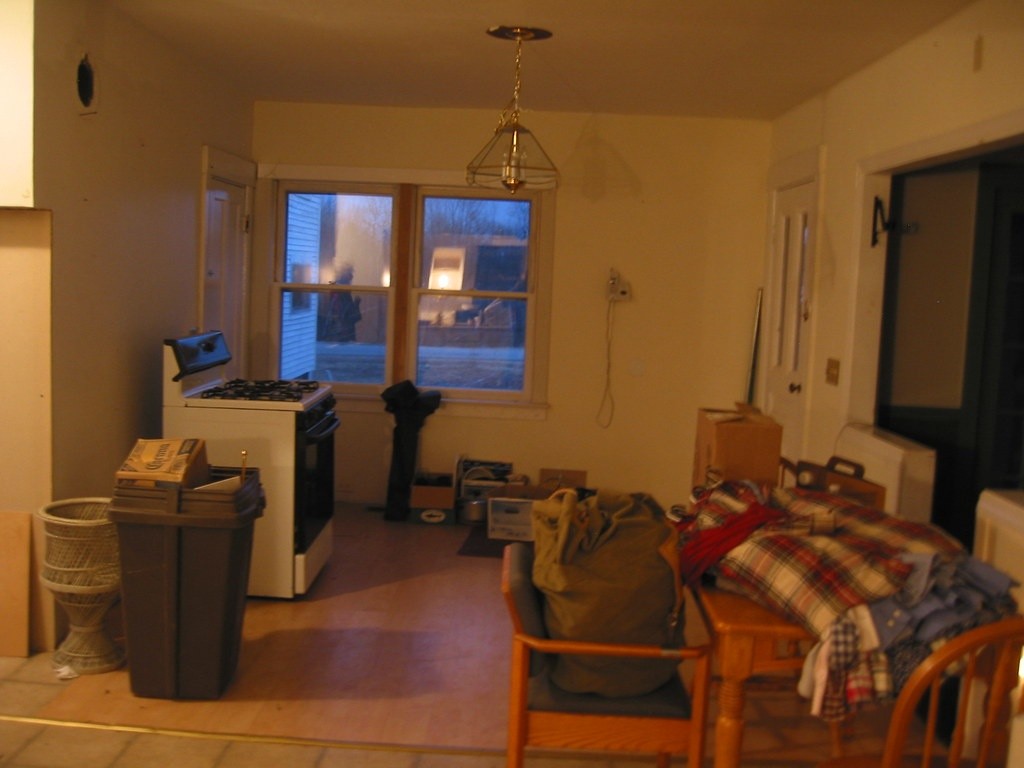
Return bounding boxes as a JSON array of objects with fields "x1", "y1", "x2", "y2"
[{"x1": 106, "y1": 468, "x2": 269, "y2": 698}]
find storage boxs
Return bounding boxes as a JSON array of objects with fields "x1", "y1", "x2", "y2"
[
  {"x1": 693, "y1": 402, "x2": 782, "y2": 489},
  {"x1": 410, "y1": 470, "x2": 455, "y2": 525},
  {"x1": 460, "y1": 480, "x2": 553, "y2": 541},
  {"x1": 116, "y1": 438, "x2": 209, "y2": 489},
  {"x1": 539, "y1": 468, "x2": 587, "y2": 492}
]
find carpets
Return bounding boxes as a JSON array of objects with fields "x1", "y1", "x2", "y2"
[{"x1": 455, "y1": 524, "x2": 508, "y2": 559}]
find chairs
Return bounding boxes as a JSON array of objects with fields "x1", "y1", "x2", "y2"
[
  {"x1": 816, "y1": 618, "x2": 1024, "y2": 768},
  {"x1": 500, "y1": 543, "x2": 712, "y2": 768}
]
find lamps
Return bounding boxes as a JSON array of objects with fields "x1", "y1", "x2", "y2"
[{"x1": 467, "y1": 25, "x2": 561, "y2": 195}]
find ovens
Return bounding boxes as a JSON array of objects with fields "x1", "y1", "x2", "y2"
[{"x1": 161, "y1": 392, "x2": 341, "y2": 599}]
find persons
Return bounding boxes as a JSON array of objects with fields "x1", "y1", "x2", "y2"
[{"x1": 322, "y1": 262, "x2": 360, "y2": 342}]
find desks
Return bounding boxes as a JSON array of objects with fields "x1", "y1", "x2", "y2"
[{"x1": 675, "y1": 531, "x2": 1024, "y2": 768}]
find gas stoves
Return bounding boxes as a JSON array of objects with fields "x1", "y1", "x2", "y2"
[{"x1": 161, "y1": 330, "x2": 333, "y2": 413}]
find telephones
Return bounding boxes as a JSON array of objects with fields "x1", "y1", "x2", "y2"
[{"x1": 606, "y1": 268, "x2": 631, "y2": 304}]
